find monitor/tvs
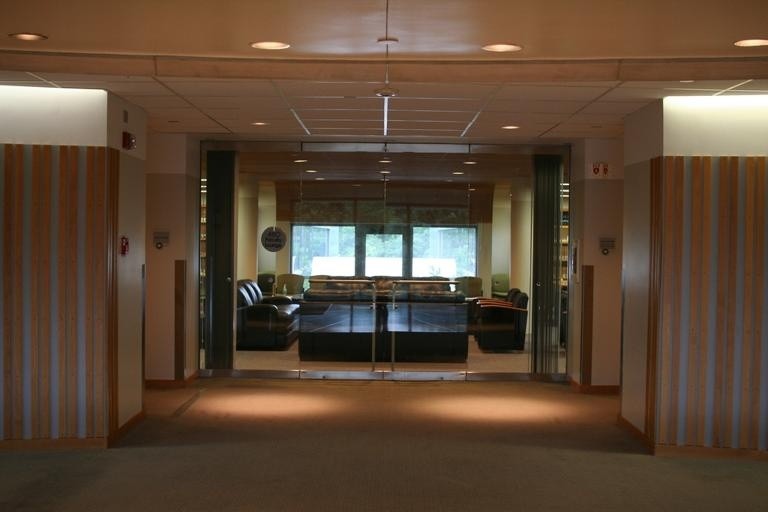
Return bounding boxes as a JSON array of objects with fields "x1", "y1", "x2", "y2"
[{"x1": 257, "y1": 272, "x2": 276, "y2": 295}]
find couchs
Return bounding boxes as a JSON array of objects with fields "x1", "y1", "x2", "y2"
[
  {"x1": 235, "y1": 278, "x2": 300, "y2": 351},
  {"x1": 303, "y1": 275, "x2": 466, "y2": 302}
]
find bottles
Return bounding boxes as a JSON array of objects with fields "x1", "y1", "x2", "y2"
[{"x1": 271, "y1": 283, "x2": 277, "y2": 298}]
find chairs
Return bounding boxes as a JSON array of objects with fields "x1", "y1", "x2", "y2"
[
  {"x1": 258, "y1": 274, "x2": 304, "y2": 304},
  {"x1": 455, "y1": 273, "x2": 509, "y2": 323},
  {"x1": 472, "y1": 288, "x2": 528, "y2": 354}
]
